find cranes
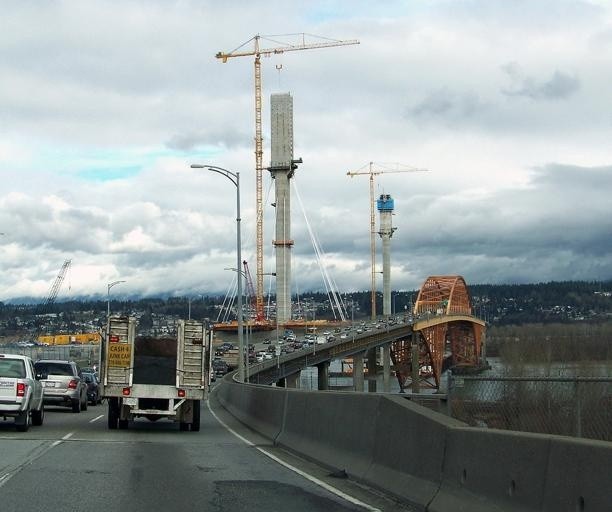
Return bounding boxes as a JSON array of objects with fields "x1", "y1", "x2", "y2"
[
  {"x1": 18, "y1": 258, "x2": 72, "y2": 341},
  {"x1": 347, "y1": 161, "x2": 429, "y2": 324},
  {"x1": 214, "y1": 34, "x2": 361, "y2": 325}
]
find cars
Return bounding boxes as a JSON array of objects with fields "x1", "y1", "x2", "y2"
[
  {"x1": 2, "y1": 340, "x2": 50, "y2": 348},
  {"x1": 211, "y1": 316, "x2": 383, "y2": 382}
]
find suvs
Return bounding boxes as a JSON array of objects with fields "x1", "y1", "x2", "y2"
[{"x1": 0, "y1": 354, "x2": 102, "y2": 431}]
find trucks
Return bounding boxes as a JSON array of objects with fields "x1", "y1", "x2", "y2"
[{"x1": 101, "y1": 315, "x2": 212, "y2": 432}]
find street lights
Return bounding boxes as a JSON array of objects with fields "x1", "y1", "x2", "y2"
[
  {"x1": 344, "y1": 292, "x2": 415, "y2": 322},
  {"x1": 106, "y1": 280, "x2": 127, "y2": 316},
  {"x1": 188, "y1": 294, "x2": 202, "y2": 319},
  {"x1": 191, "y1": 163, "x2": 249, "y2": 383},
  {"x1": 302, "y1": 297, "x2": 316, "y2": 357}
]
